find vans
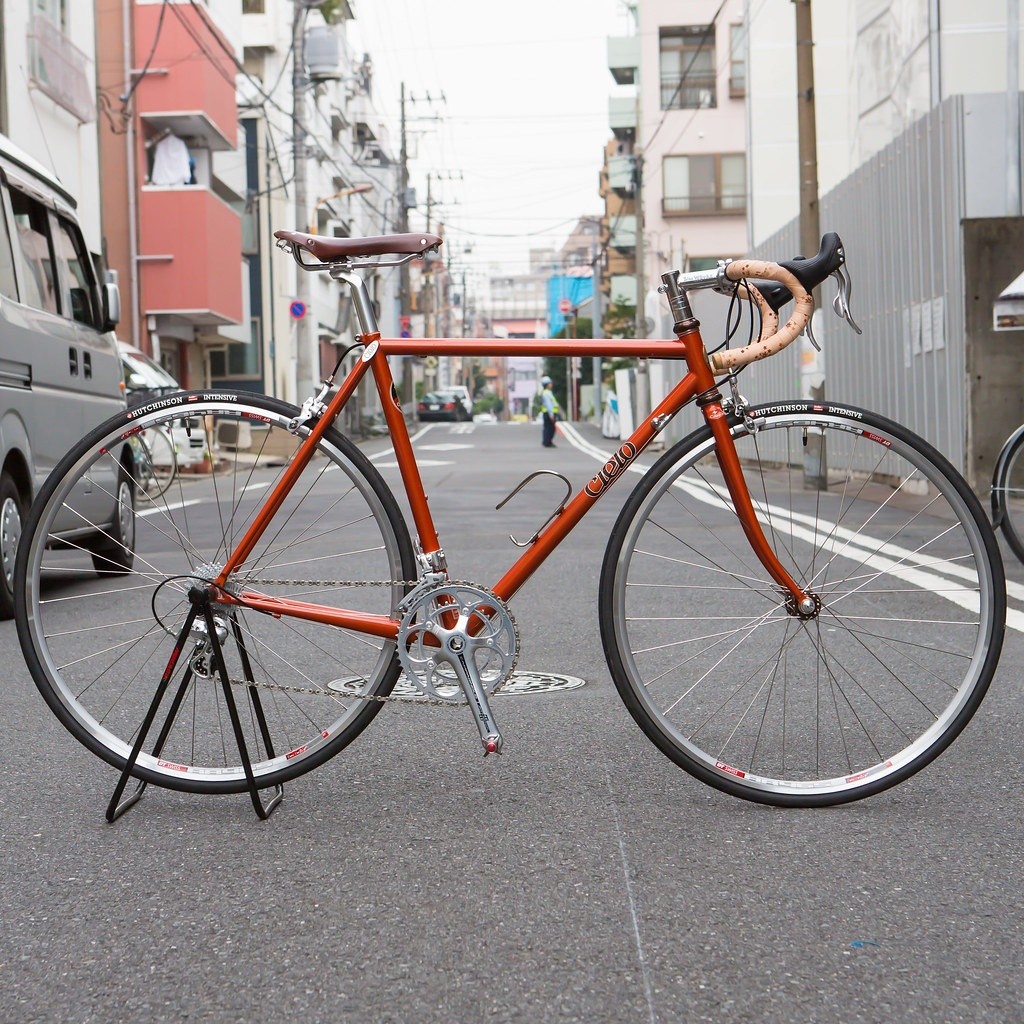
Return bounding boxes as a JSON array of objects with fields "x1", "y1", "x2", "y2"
[
  {"x1": 0, "y1": 132, "x2": 136, "y2": 620},
  {"x1": 116, "y1": 339, "x2": 206, "y2": 470}
]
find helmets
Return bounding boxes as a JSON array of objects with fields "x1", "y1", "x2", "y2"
[{"x1": 542, "y1": 376, "x2": 551, "y2": 385}]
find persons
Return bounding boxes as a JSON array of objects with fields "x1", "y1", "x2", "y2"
[{"x1": 540, "y1": 375, "x2": 560, "y2": 446}]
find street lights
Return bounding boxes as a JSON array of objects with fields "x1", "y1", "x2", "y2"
[
  {"x1": 398, "y1": 267, "x2": 446, "y2": 434},
  {"x1": 443, "y1": 248, "x2": 472, "y2": 387},
  {"x1": 286, "y1": 181, "x2": 373, "y2": 403}
]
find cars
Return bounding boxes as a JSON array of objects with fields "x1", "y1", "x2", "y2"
[
  {"x1": 416, "y1": 392, "x2": 468, "y2": 422},
  {"x1": 438, "y1": 385, "x2": 474, "y2": 420}
]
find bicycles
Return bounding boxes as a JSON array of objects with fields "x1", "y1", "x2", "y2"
[
  {"x1": 15, "y1": 226, "x2": 1007, "y2": 819},
  {"x1": 990, "y1": 423, "x2": 1024, "y2": 562}
]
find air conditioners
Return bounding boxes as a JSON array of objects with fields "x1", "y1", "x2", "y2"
[{"x1": 213, "y1": 418, "x2": 252, "y2": 449}]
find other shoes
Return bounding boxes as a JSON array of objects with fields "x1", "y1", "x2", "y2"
[{"x1": 548, "y1": 442, "x2": 557, "y2": 447}]
